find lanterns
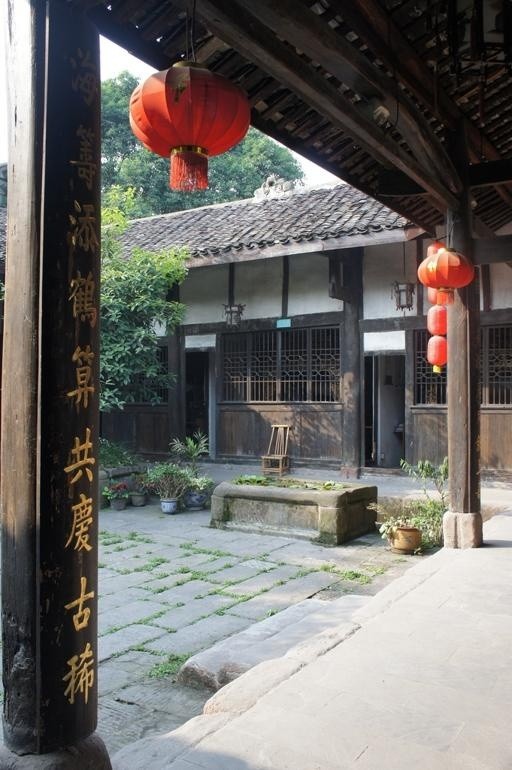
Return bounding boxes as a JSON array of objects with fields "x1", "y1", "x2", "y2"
[
  {"x1": 222, "y1": 302, "x2": 246, "y2": 331},
  {"x1": 390, "y1": 279, "x2": 419, "y2": 312},
  {"x1": 129, "y1": 61, "x2": 251, "y2": 193},
  {"x1": 415, "y1": 239, "x2": 476, "y2": 376}
]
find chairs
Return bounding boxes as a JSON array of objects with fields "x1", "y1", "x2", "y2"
[{"x1": 261, "y1": 423, "x2": 295, "y2": 477}]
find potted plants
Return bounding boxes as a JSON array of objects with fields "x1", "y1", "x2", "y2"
[
  {"x1": 101, "y1": 428, "x2": 213, "y2": 513},
  {"x1": 368, "y1": 501, "x2": 422, "y2": 554}
]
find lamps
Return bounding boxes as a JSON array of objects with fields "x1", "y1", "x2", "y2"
[
  {"x1": 392, "y1": 242, "x2": 415, "y2": 311},
  {"x1": 220, "y1": 264, "x2": 246, "y2": 328}
]
